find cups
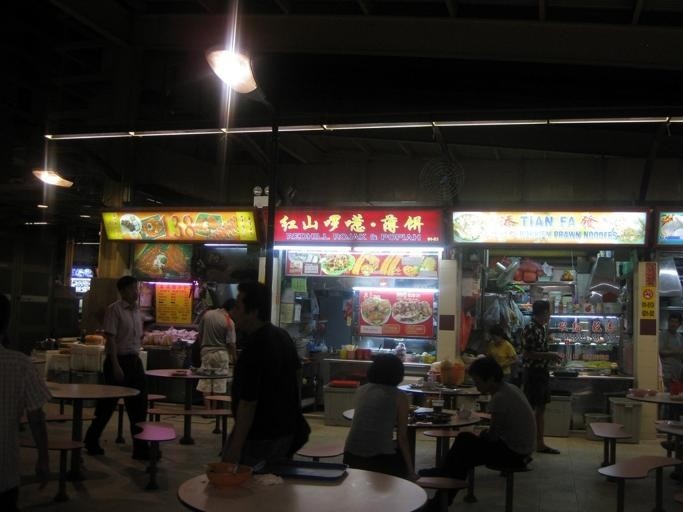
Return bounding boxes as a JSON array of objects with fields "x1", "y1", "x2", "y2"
[
  {"x1": 32, "y1": 361, "x2": 45, "y2": 378},
  {"x1": 669, "y1": 383, "x2": 680, "y2": 396},
  {"x1": 405, "y1": 399, "x2": 471, "y2": 423},
  {"x1": 143, "y1": 335, "x2": 171, "y2": 345},
  {"x1": 335, "y1": 349, "x2": 370, "y2": 360}
]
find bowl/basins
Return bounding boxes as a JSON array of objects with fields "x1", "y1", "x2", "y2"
[
  {"x1": 40, "y1": 342, "x2": 69, "y2": 350},
  {"x1": 69, "y1": 343, "x2": 103, "y2": 374}
]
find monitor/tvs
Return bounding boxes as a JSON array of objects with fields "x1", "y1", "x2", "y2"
[{"x1": 71, "y1": 266, "x2": 95, "y2": 296}]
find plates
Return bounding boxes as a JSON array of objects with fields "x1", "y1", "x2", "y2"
[
  {"x1": 318, "y1": 253, "x2": 354, "y2": 275},
  {"x1": 629, "y1": 388, "x2": 659, "y2": 397},
  {"x1": 119, "y1": 214, "x2": 143, "y2": 234},
  {"x1": 360, "y1": 297, "x2": 390, "y2": 326},
  {"x1": 390, "y1": 300, "x2": 431, "y2": 325}
]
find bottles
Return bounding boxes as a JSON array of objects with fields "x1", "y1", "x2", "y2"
[
  {"x1": 79, "y1": 329, "x2": 86, "y2": 344},
  {"x1": 409, "y1": 361, "x2": 474, "y2": 389},
  {"x1": 411, "y1": 350, "x2": 437, "y2": 363}
]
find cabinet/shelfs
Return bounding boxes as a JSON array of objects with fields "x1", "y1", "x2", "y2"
[{"x1": 520, "y1": 309, "x2": 624, "y2": 365}]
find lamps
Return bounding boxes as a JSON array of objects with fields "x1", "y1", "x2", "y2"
[
  {"x1": 202, "y1": 43, "x2": 258, "y2": 94},
  {"x1": 31, "y1": 169, "x2": 74, "y2": 189},
  {"x1": 252, "y1": 184, "x2": 270, "y2": 209}
]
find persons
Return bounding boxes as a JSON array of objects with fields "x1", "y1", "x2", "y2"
[
  {"x1": 414, "y1": 300, "x2": 562, "y2": 512},
  {"x1": 342, "y1": 353, "x2": 420, "y2": 486},
  {"x1": 81, "y1": 274, "x2": 161, "y2": 462},
  {"x1": 0, "y1": 291, "x2": 53, "y2": 512},
  {"x1": 192, "y1": 278, "x2": 311, "y2": 477},
  {"x1": 658, "y1": 312, "x2": 683, "y2": 423}
]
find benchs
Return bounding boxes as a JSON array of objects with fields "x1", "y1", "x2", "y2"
[{"x1": 11, "y1": 331, "x2": 682, "y2": 512}]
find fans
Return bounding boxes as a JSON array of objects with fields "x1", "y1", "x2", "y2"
[{"x1": 492, "y1": 260, "x2": 521, "y2": 288}]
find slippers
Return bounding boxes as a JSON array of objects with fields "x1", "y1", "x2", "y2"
[{"x1": 536, "y1": 447, "x2": 559, "y2": 455}]
